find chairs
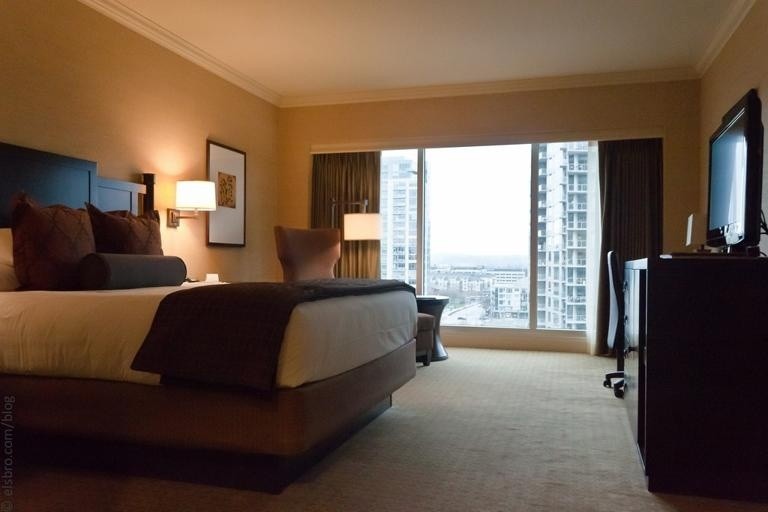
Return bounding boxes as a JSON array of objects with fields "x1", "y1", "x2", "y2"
[
  {"x1": 273, "y1": 225, "x2": 341, "y2": 282},
  {"x1": 603, "y1": 251, "x2": 627, "y2": 398}
]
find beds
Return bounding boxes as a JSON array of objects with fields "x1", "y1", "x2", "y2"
[{"x1": 0, "y1": 141, "x2": 420, "y2": 496}]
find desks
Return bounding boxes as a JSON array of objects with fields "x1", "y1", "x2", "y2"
[{"x1": 415, "y1": 295, "x2": 449, "y2": 362}]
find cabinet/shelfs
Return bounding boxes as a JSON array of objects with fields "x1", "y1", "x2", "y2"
[{"x1": 622, "y1": 258, "x2": 767, "y2": 504}]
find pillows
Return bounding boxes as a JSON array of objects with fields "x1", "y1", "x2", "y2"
[{"x1": 0, "y1": 189, "x2": 187, "y2": 292}]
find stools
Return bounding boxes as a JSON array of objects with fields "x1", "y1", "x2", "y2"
[{"x1": 416, "y1": 313, "x2": 434, "y2": 365}]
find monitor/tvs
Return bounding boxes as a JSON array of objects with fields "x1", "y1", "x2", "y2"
[{"x1": 705, "y1": 88, "x2": 765, "y2": 256}]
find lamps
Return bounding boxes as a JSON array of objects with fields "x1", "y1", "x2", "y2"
[
  {"x1": 167, "y1": 180, "x2": 216, "y2": 227},
  {"x1": 329, "y1": 196, "x2": 383, "y2": 280}
]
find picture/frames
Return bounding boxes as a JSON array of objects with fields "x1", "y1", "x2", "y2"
[{"x1": 206, "y1": 139, "x2": 246, "y2": 247}]
